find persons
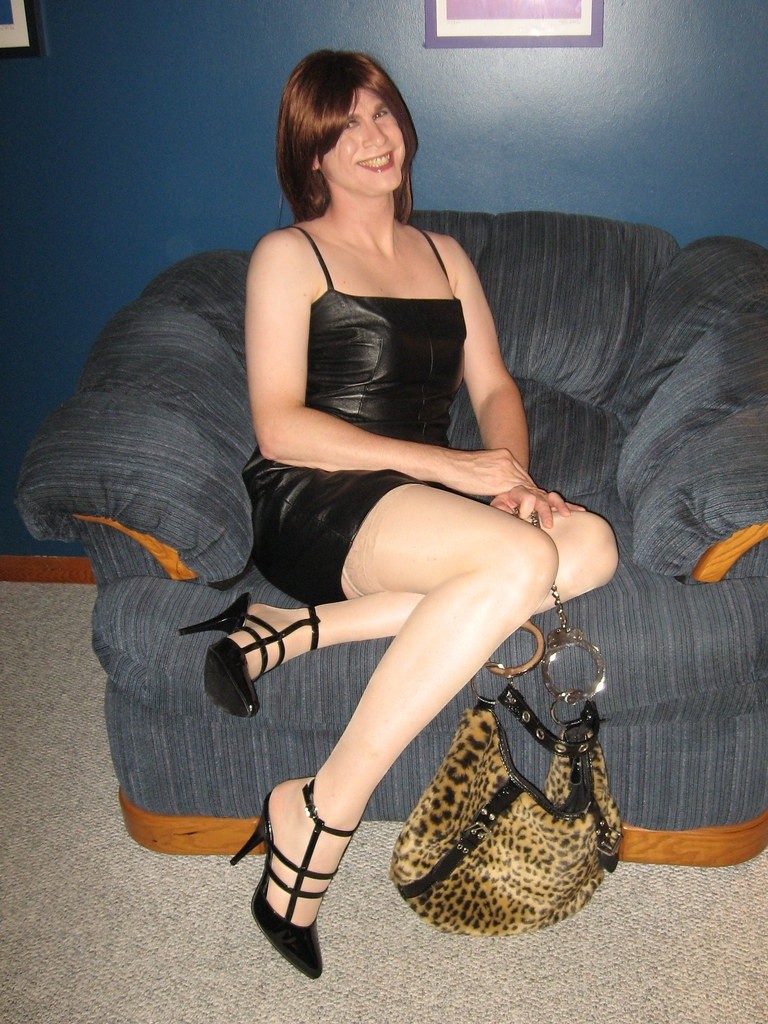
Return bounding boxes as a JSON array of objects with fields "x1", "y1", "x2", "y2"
[{"x1": 177, "y1": 46, "x2": 619, "y2": 978}]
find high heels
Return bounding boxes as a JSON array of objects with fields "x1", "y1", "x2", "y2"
[
  {"x1": 179, "y1": 592, "x2": 322, "y2": 720},
  {"x1": 229, "y1": 776, "x2": 356, "y2": 980}
]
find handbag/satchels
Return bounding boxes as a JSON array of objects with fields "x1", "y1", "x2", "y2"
[{"x1": 389, "y1": 504, "x2": 622, "y2": 937}]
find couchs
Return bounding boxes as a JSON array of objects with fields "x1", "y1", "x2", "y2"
[{"x1": 14, "y1": 207, "x2": 767, "y2": 870}]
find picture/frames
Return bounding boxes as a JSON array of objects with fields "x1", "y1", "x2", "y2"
[
  {"x1": 0, "y1": 0, "x2": 46, "y2": 58},
  {"x1": 424, "y1": 0, "x2": 603, "y2": 47}
]
